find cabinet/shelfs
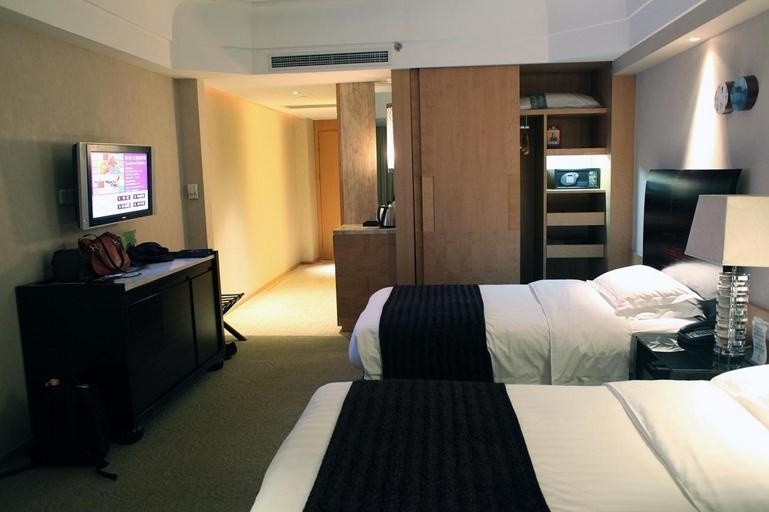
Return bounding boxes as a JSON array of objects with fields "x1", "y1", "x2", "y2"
[
  {"x1": 520, "y1": 64, "x2": 609, "y2": 284},
  {"x1": 332, "y1": 220, "x2": 396, "y2": 333},
  {"x1": 16, "y1": 248, "x2": 226, "y2": 450}
]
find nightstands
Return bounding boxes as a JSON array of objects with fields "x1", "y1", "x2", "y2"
[{"x1": 629, "y1": 334, "x2": 756, "y2": 379}]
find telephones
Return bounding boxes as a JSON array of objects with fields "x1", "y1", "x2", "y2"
[{"x1": 678, "y1": 322, "x2": 714, "y2": 351}]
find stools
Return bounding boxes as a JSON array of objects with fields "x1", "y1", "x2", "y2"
[{"x1": 220, "y1": 293, "x2": 250, "y2": 342}]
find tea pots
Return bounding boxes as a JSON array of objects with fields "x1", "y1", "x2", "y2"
[{"x1": 376, "y1": 198, "x2": 395, "y2": 228}]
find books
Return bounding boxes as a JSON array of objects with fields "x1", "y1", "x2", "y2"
[{"x1": 638, "y1": 335, "x2": 686, "y2": 353}]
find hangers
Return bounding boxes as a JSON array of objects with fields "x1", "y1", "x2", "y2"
[{"x1": 519, "y1": 126, "x2": 532, "y2": 156}]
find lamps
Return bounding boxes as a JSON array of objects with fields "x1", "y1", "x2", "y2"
[
  {"x1": 682, "y1": 192, "x2": 768, "y2": 359},
  {"x1": 384, "y1": 103, "x2": 396, "y2": 174}
]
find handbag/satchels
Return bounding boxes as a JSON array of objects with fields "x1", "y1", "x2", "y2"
[{"x1": 76, "y1": 232, "x2": 132, "y2": 281}]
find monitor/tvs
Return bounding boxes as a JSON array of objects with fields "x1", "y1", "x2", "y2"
[{"x1": 72, "y1": 142, "x2": 156, "y2": 231}]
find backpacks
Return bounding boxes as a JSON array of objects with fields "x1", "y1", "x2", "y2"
[{"x1": 31, "y1": 377, "x2": 111, "y2": 466}]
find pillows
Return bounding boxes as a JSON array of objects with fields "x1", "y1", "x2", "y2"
[
  {"x1": 712, "y1": 362, "x2": 769, "y2": 428},
  {"x1": 519, "y1": 92, "x2": 601, "y2": 109},
  {"x1": 624, "y1": 301, "x2": 709, "y2": 320},
  {"x1": 589, "y1": 264, "x2": 706, "y2": 311}
]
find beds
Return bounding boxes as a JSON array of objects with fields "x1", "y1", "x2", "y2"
[
  {"x1": 347, "y1": 168, "x2": 746, "y2": 387},
  {"x1": 247, "y1": 362, "x2": 769, "y2": 512}
]
